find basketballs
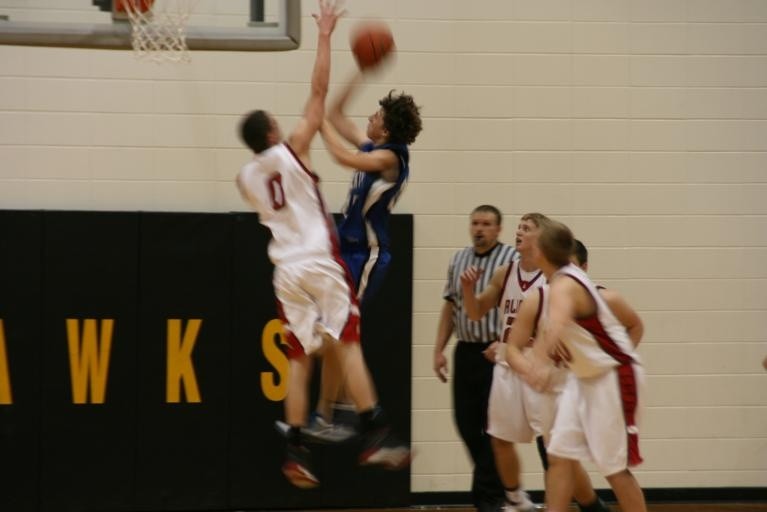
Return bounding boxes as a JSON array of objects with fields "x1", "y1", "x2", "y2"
[{"x1": 352, "y1": 26, "x2": 394, "y2": 70}]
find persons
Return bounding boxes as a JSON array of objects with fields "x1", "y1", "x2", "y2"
[
  {"x1": 504, "y1": 283, "x2": 611, "y2": 510},
  {"x1": 433, "y1": 204, "x2": 521, "y2": 511},
  {"x1": 520, "y1": 218, "x2": 650, "y2": 512},
  {"x1": 273, "y1": 71, "x2": 425, "y2": 443},
  {"x1": 762, "y1": 354, "x2": 766, "y2": 370},
  {"x1": 234, "y1": 1, "x2": 416, "y2": 488},
  {"x1": 562, "y1": 239, "x2": 643, "y2": 510},
  {"x1": 460, "y1": 213, "x2": 557, "y2": 512}
]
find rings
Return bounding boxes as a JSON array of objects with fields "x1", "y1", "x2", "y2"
[{"x1": 551, "y1": 346, "x2": 556, "y2": 354}]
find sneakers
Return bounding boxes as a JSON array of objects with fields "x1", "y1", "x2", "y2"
[
  {"x1": 272, "y1": 407, "x2": 418, "y2": 489},
  {"x1": 501, "y1": 497, "x2": 535, "y2": 512}
]
